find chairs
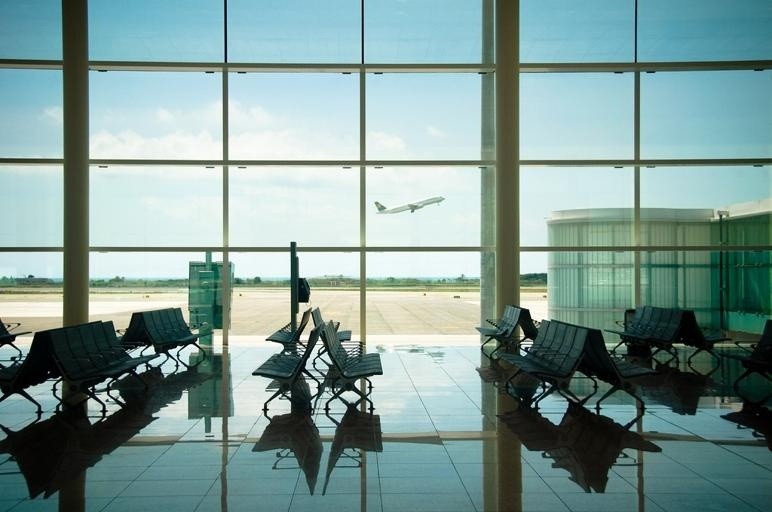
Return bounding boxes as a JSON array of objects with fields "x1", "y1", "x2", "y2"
[
  {"x1": 252, "y1": 363, "x2": 383, "y2": 496},
  {"x1": 252, "y1": 307, "x2": 383, "y2": 410},
  {"x1": 476, "y1": 347, "x2": 772, "y2": 493},
  {"x1": 94, "y1": 367, "x2": 216, "y2": 413},
  {"x1": 474, "y1": 305, "x2": 772, "y2": 409},
  {"x1": 115, "y1": 307, "x2": 214, "y2": 365},
  {"x1": 1, "y1": 320, "x2": 160, "y2": 419},
  {"x1": 0, "y1": 414, "x2": 160, "y2": 499}
]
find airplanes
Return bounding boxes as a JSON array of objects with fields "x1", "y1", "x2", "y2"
[{"x1": 375, "y1": 196, "x2": 444, "y2": 214}]
